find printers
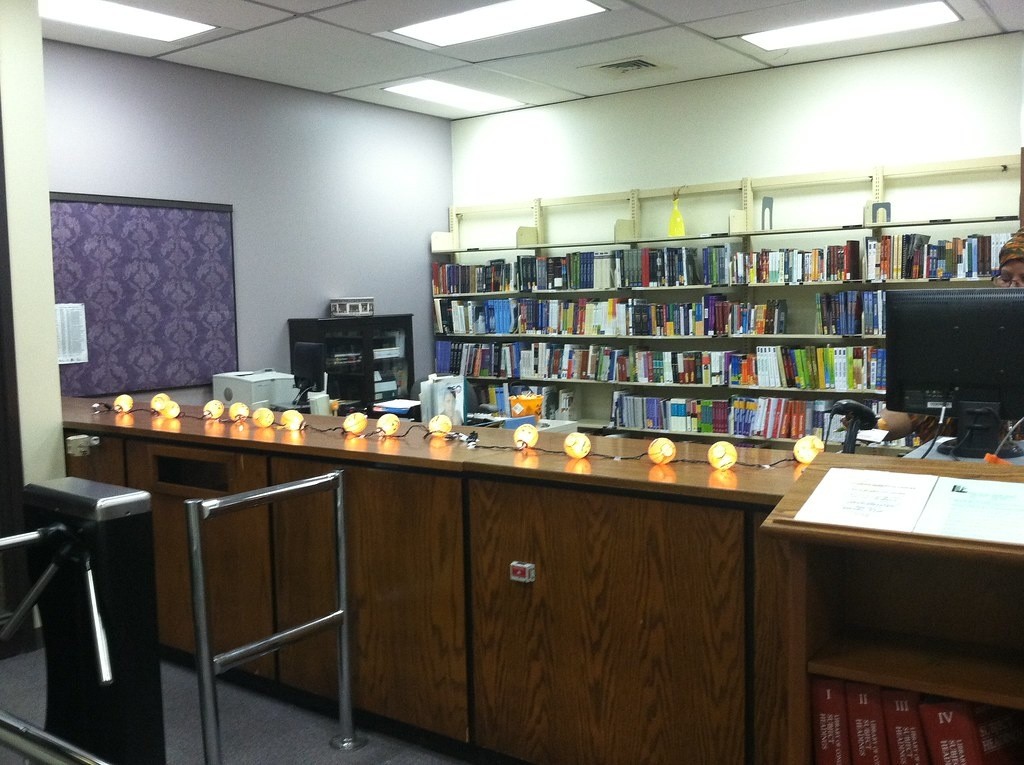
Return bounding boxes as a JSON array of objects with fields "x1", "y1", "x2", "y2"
[{"x1": 212, "y1": 368, "x2": 302, "y2": 411}]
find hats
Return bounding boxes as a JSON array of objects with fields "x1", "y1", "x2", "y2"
[
  {"x1": 999, "y1": 227, "x2": 1024, "y2": 273},
  {"x1": 991, "y1": 273, "x2": 1024, "y2": 288}
]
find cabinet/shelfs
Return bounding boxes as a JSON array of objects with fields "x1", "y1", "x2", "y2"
[
  {"x1": 286, "y1": 313, "x2": 415, "y2": 418},
  {"x1": 762, "y1": 447, "x2": 1022, "y2": 762},
  {"x1": 431, "y1": 235, "x2": 993, "y2": 444},
  {"x1": 61, "y1": 396, "x2": 807, "y2": 765}
]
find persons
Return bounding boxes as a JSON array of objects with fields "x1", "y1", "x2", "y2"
[
  {"x1": 840, "y1": 226, "x2": 1024, "y2": 441},
  {"x1": 441, "y1": 387, "x2": 463, "y2": 425}
]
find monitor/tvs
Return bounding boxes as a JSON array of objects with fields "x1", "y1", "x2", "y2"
[{"x1": 885, "y1": 287, "x2": 1024, "y2": 458}]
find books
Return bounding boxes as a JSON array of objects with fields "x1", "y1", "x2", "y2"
[
  {"x1": 431, "y1": 232, "x2": 1015, "y2": 446},
  {"x1": 419, "y1": 375, "x2": 464, "y2": 426}
]
